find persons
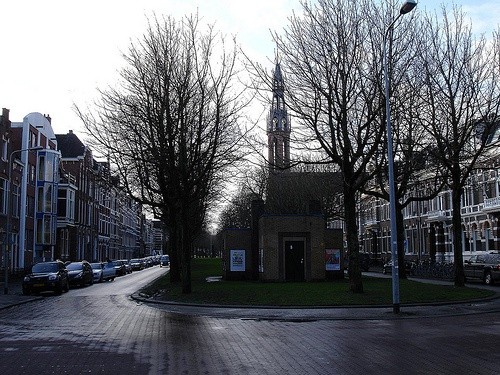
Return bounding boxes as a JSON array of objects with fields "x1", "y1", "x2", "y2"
[
  {"x1": 411, "y1": 257, "x2": 420, "y2": 272},
  {"x1": 327, "y1": 254, "x2": 336, "y2": 264}
]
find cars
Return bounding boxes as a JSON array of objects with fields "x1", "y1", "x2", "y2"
[
  {"x1": 463, "y1": 253, "x2": 500, "y2": 286},
  {"x1": 64, "y1": 261, "x2": 94, "y2": 289},
  {"x1": 111, "y1": 255, "x2": 162, "y2": 277},
  {"x1": 383, "y1": 259, "x2": 416, "y2": 275},
  {"x1": 89, "y1": 263, "x2": 116, "y2": 284}
]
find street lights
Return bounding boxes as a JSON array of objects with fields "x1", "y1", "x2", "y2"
[
  {"x1": 4, "y1": 146, "x2": 44, "y2": 294},
  {"x1": 381, "y1": 0, "x2": 419, "y2": 313}
]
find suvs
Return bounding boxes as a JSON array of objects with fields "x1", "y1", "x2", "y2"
[
  {"x1": 159, "y1": 255, "x2": 170, "y2": 268},
  {"x1": 23, "y1": 259, "x2": 69, "y2": 296}
]
